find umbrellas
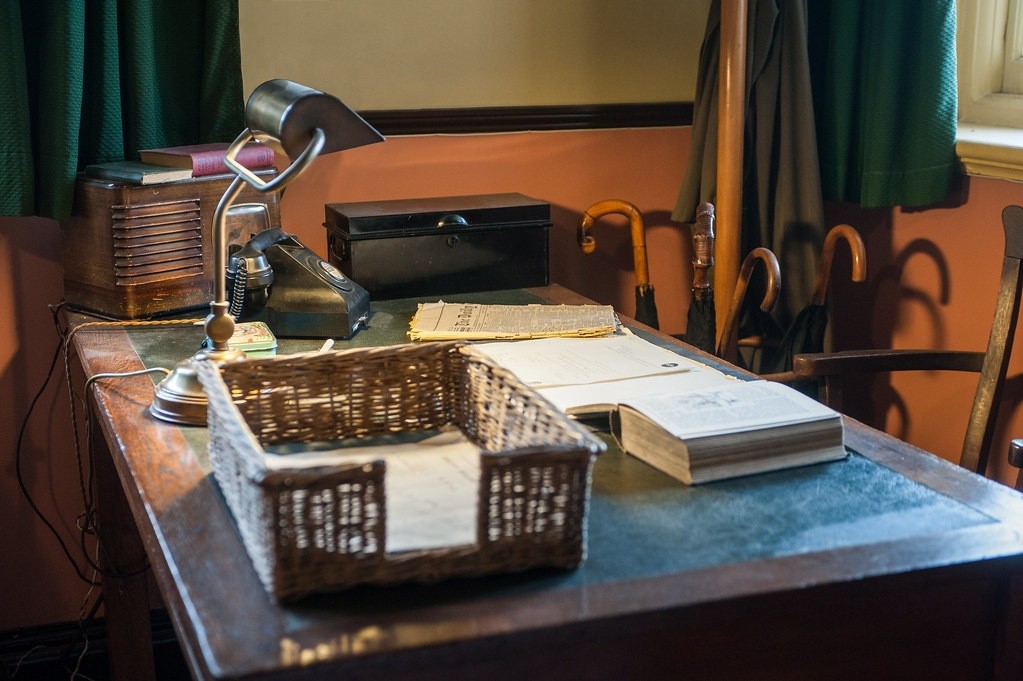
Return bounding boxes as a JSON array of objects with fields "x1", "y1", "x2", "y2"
[
  {"x1": 578, "y1": 200, "x2": 659, "y2": 331},
  {"x1": 682, "y1": 200, "x2": 715, "y2": 355},
  {"x1": 719, "y1": 223, "x2": 867, "y2": 400}
]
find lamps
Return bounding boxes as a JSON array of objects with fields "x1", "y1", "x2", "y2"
[{"x1": 149, "y1": 78, "x2": 386, "y2": 427}]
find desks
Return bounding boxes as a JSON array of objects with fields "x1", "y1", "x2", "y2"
[{"x1": 66, "y1": 282, "x2": 1022, "y2": 681}]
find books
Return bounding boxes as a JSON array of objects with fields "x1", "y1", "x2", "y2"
[
  {"x1": 564, "y1": 372, "x2": 850, "y2": 484},
  {"x1": 138, "y1": 143, "x2": 274, "y2": 176},
  {"x1": 86, "y1": 160, "x2": 194, "y2": 184}
]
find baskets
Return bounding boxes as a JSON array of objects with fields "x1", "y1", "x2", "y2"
[{"x1": 193, "y1": 339, "x2": 607, "y2": 603}]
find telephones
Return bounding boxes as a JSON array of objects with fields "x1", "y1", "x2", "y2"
[{"x1": 224, "y1": 224, "x2": 372, "y2": 340}]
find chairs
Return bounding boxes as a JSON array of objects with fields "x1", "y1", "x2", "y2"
[{"x1": 792, "y1": 204, "x2": 1023, "y2": 493}]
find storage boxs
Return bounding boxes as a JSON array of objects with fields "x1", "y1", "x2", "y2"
[
  {"x1": 322, "y1": 192, "x2": 552, "y2": 301},
  {"x1": 228, "y1": 321, "x2": 278, "y2": 359}
]
